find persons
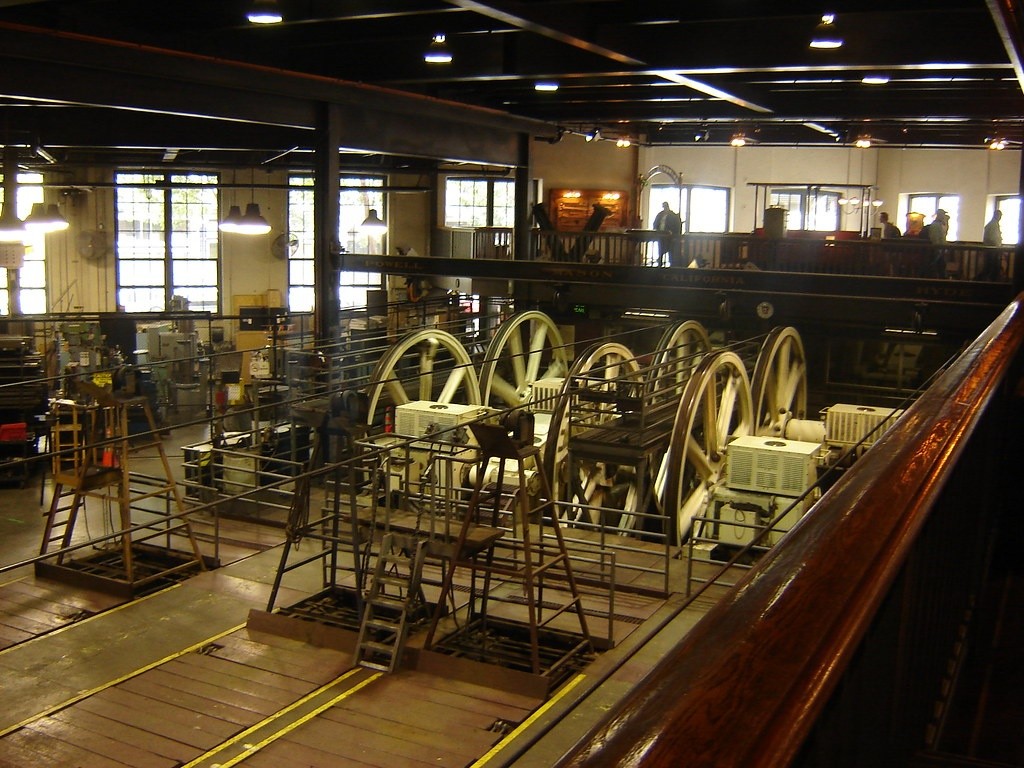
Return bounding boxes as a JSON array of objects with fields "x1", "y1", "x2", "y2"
[
  {"x1": 879, "y1": 212, "x2": 901, "y2": 239},
  {"x1": 929, "y1": 209, "x2": 947, "y2": 244},
  {"x1": 984, "y1": 210, "x2": 1004, "y2": 247},
  {"x1": 653, "y1": 203, "x2": 682, "y2": 258}
]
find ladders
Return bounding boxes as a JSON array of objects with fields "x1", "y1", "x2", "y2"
[
  {"x1": 350, "y1": 531, "x2": 428, "y2": 674},
  {"x1": 37, "y1": 482, "x2": 82, "y2": 564},
  {"x1": 50, "y1": 402, "x2": 83, "y2": 476}
]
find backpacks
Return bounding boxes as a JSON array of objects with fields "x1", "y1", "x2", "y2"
[
  {"x1": 918, "y1": 224, "x2": 929, "y2": 239},
  {"x1": 887, "y1": 225, "x2": 900, "y2": 238}
]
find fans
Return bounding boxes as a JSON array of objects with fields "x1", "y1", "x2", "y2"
[
  {"x1": 73, "y1": 230, "x2": 107, "y2": 260},
  {"x1": 270, "y1": 232, "x2": 300, "y2": 260}
]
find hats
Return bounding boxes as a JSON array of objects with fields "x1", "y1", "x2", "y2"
[{"x1": 936, "y1": 209, "x2": 947, "y2": 214}]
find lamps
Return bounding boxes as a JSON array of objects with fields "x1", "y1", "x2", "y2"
[
  {"x1": 584, "y1": 127, "x2": 600, "y2": 143},
  {"x1": 809, "y1": 23, "x2": 843, "y2": 50},
  {"x1": 422, "y1": 39, "x2": 453, "y2": 63},
  {"x1": 359, "y1": 207, "x2": 387, "y2": 236},
  {"x1": 855, "y1": 133, "x2": 872, "y2": 148},
  {"x1": 729, "y1": 132, "x2": 746, "y2": 148},
  {"x1": 0, "y1": 201, "x2": 70, "y2": 242},
  {"x1": 988, "y1": 135, "x2": 1009, "y2": 150},
  {"x1": 838, "y1": 146, "x2": 883, "y2": 216},
  {"x1": 219, "y1": 170, "x2": 272, "y2": 236}
]
